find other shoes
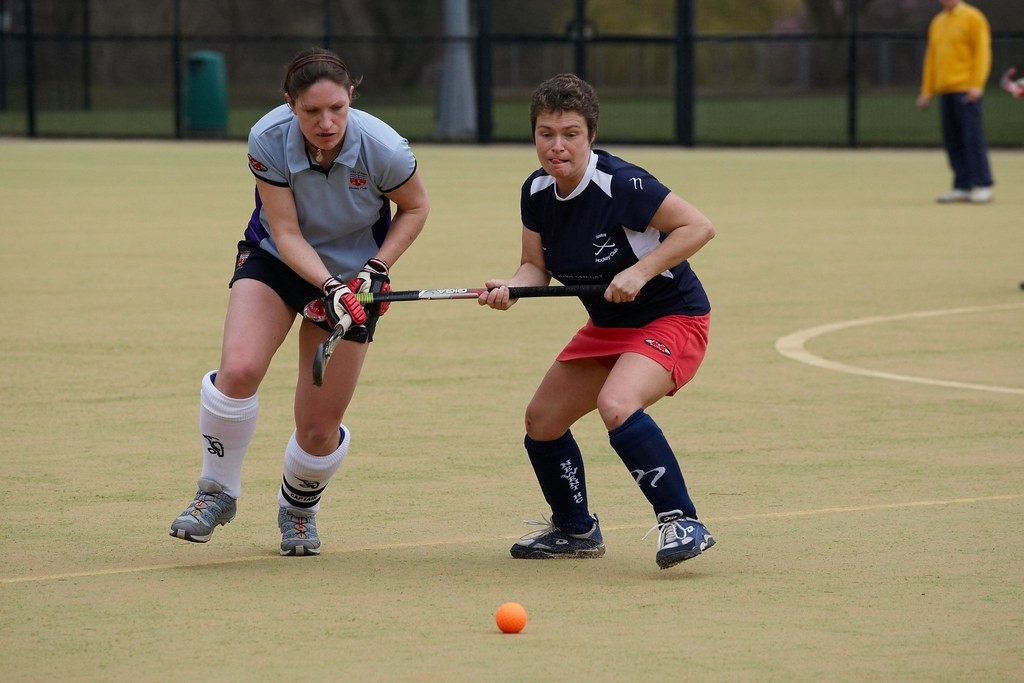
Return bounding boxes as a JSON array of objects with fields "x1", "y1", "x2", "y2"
[
  {"x1": 938, "y1": 189, "x2": 970, "y2": 203},
  {"x1": 969, "y1": 186, "x2": 994, "y2": 202}
]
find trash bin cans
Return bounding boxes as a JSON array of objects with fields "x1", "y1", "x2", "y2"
[{"x1": 179, "y1": 52, "x2": 231, "y2": 137}]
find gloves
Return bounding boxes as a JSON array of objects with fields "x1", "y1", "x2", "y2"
[
  {"x1": 357, "y1": 258, "x2": 390, "y2": 316},
  {"x1": 322, "y1": 276, "x2": 367, "y2": 331}
]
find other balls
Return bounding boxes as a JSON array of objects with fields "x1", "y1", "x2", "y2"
[{"x1": 496, "y1": 603, "x2": 528, "y2": 635}]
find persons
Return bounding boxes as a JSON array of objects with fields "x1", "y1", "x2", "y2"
[
  {"x1": 169, "y1": 47, "x2": 429, "y2": 557},
  {"x1": 477, "y1": 74, "x2": 718, "y2": 569},
  {"x1": 916, "y1": 0, "x2": 996, "y2": 205}
]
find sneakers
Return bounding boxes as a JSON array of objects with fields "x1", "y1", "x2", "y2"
[
  {"x1": 278, "y1": 507, "x2": 322, "y2": 556},
  {"x1": 641, "y1": 509, "x2": 716, "y2": 568},
  {"x1": 510, "y1": 510, "x2": 605, "y2": 559},
  {"x1": 169, "y1": 478, "x2": 237, "y2": 543}
]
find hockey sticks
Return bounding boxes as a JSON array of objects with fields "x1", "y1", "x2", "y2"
[
  {"x1": 312, "y1": 310, "x2": 354, "y2": 388},
  {"x1": 304, "y1": 281, "x2": 641, "y2": 321}
]
